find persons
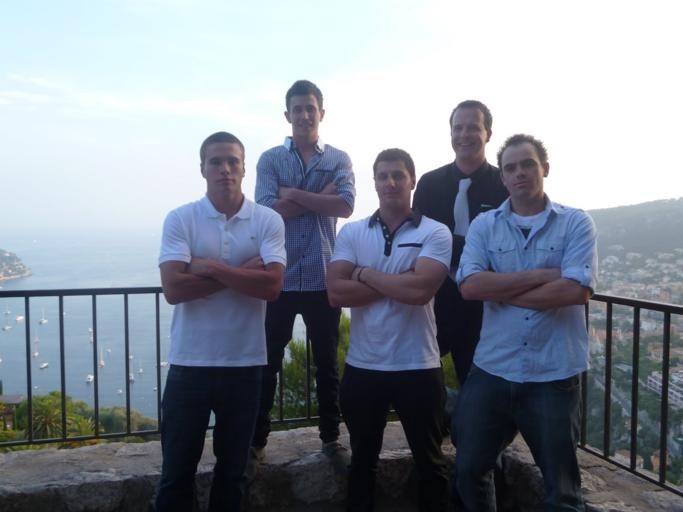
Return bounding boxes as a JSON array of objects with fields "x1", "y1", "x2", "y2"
[
  {"x1": 326, "y1": 148, "x2": 452, "y2": 510},
  {"x1": 411, "y1": 101, "x2": 511, "y2": 511},
  {"x1": 155, "y1": 132, "x2": 286, "y2": 512},
  {"x1": 246, "y1": 79, "x2": 355, "y2": 474},
  {"x1": 450, "y1": 134, "x2": 598, "y2": 511}
]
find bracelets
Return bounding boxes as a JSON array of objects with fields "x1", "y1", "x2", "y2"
[{"x1": 357, "y1": 264, "x2": 367, "y2": 282}]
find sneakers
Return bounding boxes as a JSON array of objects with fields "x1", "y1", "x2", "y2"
[
  {"x1": 241, "y1": 444, "x2": 266, "y2": 485},
  {"x1": 321, "y1": 440, "x2": 353, "y2": 473}
]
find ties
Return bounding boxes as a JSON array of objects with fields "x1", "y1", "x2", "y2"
[{"x1": 450, "y1": 176, "x2": 473, "y2": 238}]
